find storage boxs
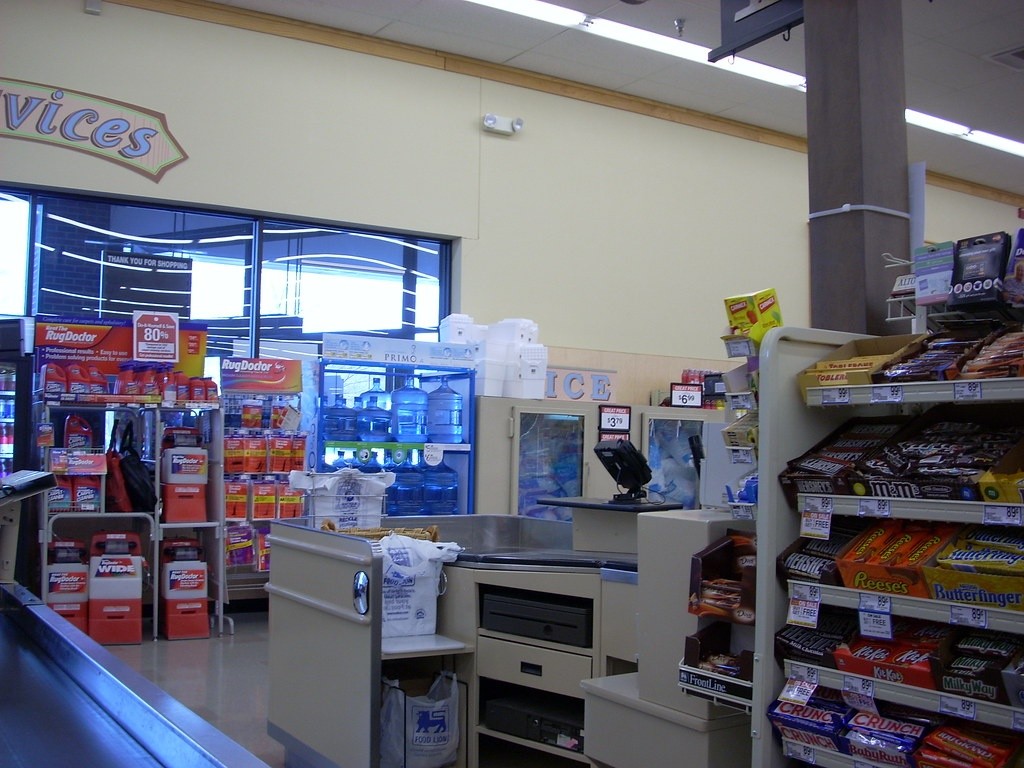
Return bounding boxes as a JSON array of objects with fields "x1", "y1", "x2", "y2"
[
  {"x1": 48, "y1": 447, "x2": 210, "y2": 645},
  {"x1": 438, "y1": 314, "x2": 549, "y2": 400},
  {"x1": 684, "y1": 230, "x2": 1024, "y2": 768}
]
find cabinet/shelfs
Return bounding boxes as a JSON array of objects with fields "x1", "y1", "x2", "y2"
[
  {"x1": 220, "y1": 388, "x2": 313, "y2": 635},
  {"x1": 40, "y1": 398, "x2": 225, "y2": 641},
  {"x1": 319, "y1": 358, "x2": 476, "y2": 516},
  {"x1": 750, "y1": 326, "x2": 1024, "y2": 768}
]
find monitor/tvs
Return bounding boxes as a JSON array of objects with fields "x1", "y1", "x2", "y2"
[{"x1": 594, "y1": 440, "x2": 653, "y2": 488}]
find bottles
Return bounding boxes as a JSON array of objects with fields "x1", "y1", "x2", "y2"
[
  {"x1": 384, "y1": 450, "x2": 425, "y2": 516},
  {"x1": 0, "y1": 369, "x2": 16, "y2": 477},
  {"x1": 428, "y1": 376, "x2": 464, "y2": 444},
  {"x1": 324, "y1": 392, "x2": 396, "y2": 475},
  {"x1": 421, "y1": 458, "x2": 460, "y2": 515},
  {"x1": 359, "y1": 377, "x2": 393, "y2": 433},
  {"x1": 416, "y1": 449, "x2": 426, "y2": 472},
  {"x1": 391, "y1": 374, "x2": 428, "y2": 443},
  {"x1": 42, "y1": 361, "x2": 219, "y2": 512}
]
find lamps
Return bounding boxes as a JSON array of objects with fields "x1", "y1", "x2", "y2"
[{"x1": 482, "y1": 113, "x2": 524, "y2": 136}]
